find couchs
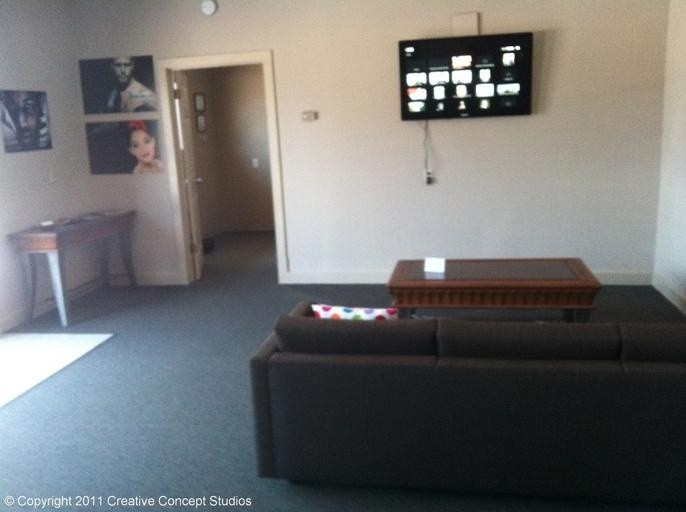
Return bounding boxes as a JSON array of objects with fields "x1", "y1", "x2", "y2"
[{"x1": 250, "y1": 301, "x2": 686, "y2": 508}]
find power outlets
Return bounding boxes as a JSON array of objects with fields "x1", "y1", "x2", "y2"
[{"x1": 424, "y1": 168, "x2": 433, "y2": 185}]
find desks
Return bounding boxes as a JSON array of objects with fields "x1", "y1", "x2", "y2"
[{"x1": 8, "y1": 208, "x2": 136, "y2": 327}]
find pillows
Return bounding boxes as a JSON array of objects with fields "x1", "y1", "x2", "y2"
[{"x1": 308, "y1": 303, "x2": 399, "y2": 322}]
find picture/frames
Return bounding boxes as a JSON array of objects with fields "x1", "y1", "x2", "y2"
[{"x1": 192, "y1": 93, "x2": 208, "y2": 133}]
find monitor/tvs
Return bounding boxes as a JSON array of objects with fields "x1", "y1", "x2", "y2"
[{"x1": 399, "y1": 33, "x2": 531, "y2": 120}]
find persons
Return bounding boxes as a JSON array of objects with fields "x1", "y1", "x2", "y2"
[
  {"x1": 4, "y1": 91, "x2": 43, "y2": 147},
  {"x1": 106, "y1": 58, "x2": 157, "y2": 112},
  {"x1": 126, "y1": 121, "x2": 164, "y2": 175}
]
